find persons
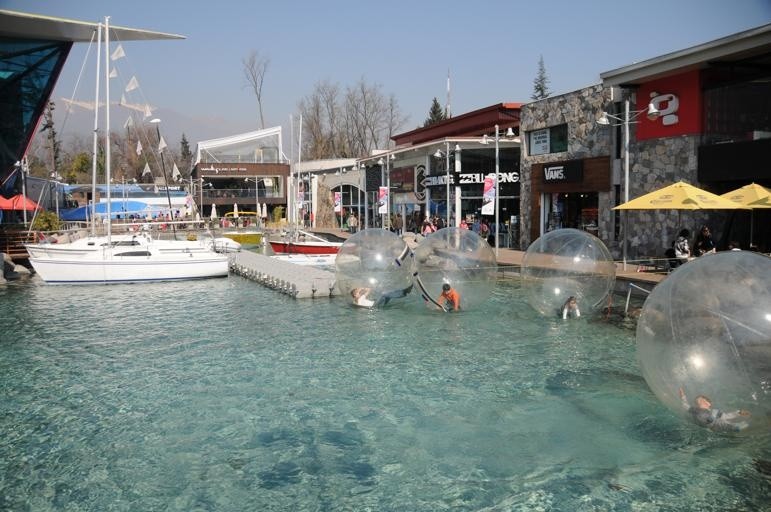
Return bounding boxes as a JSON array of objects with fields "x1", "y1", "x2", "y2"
[
  {"x1": 673, "y1": 228, "x2": 691, "y2": 257},
  {"x1": 679, "y1": 388, "x2": 750, "y2": 433},
  {"x1": 393, "y1": 211, "x2": 491, "y2": 252},
  {"x1": 198, "y1": 217, "x2": 206, "y2": 228},
  {"x1": 665, "y1": 240, "x2": 682, "y2": 267},
  {"x1": 729, "y1": 240, "x2": 741, "y2": 251},
  {"x1": 115, "y1": 206, "x2": 189, "y2": 233},
  {"x1": 347, "y1": 212, "x2": 357, "y2": 233},
  {"x1": 304, "y1": 212, "x2": 308, "y2": 228},
  {"x1": 699, "y1": 225, "x2": 715, "y2": 256},
  {"x1": 561, "y1": 295, "x2": 581, "y2": 320},
  {"x1": 435, "y1": 283, "x2": 461, "y2": 312},
  {"x1": 218, "y1": 216, "x2": 251, "y2": 228},
  {"x1": 309, "y1": 212, "x2": 314, "y2": 227},
  {"x1": 351, "y1": 284, "x2": 414, "y2": 307}
]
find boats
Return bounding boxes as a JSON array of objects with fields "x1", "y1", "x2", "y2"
[{"x1": 270, "y1": 227, "x2": 354, "y2": 256}]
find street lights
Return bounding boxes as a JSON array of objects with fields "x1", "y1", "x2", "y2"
[
  {"x1": 433, "y1": 144, "x2": 461, "y2": 249},
  {"x1": 480, "y1": 125, "x2": 515, "y2": 257},
  {"x1": 244, "y1": 178, "x2": 264, "y2": 214},
  {"x1": 597, "y1": 100, "x2": 660, "y2": 271},
  {"x1": 178, "y1": 178, "x2": 212, "y2": 218},
  {"x1": 304, "y1": 154, "x2": 395, "y2": 229}
]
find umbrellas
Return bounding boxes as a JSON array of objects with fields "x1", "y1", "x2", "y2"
[
  {"x1": 256, "y1": 203, "x2": 261, "y2": 217},
  {"x1": 8, "y1": 193, "x2": 42, "y2": 211},
  {"x1": 610, "y1": 180, "x2": 752, "y2": 230},
  {"x1": 262, "y1": 203, "x2": 267, "y2": 218},
  {"x1": 0, "y1": 194, "x2": 14, "y2": 210},
  {"x1": 234, "y1": 202, "x2": 239, "y2": 217},
  {"x1": 718, "y1": 181, "x2": 771, "y2": 246},
  {"x1": 210, "y1": 203, "x2": 219, "y2": 228}
]
